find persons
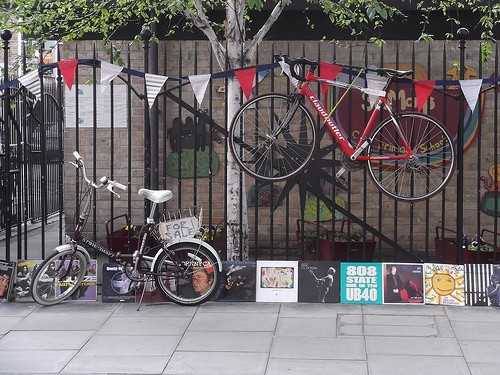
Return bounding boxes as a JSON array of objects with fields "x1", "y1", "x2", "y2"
[
  {"x1": 0, "y1": 268, "x2": 13, "y2": 297},
  {"x1": 386, "y1": 265, "x2": 403, "y2": 303},
  {"x1": 17, "y1": 261, "x2": 96, "y2": 300},
  {"x1": 316, "y1": 267, "x2": 336, "y2": 302},
  {"x1": 487, "y1": 274, "x2": 500, "y2": 307},
  {"x1": 191, "y1": 269, "x2": 214, "y2": 296}
]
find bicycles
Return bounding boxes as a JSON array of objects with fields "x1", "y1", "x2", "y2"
[
  {"x1": 31, "y1": 150, "x2": 223, "y2": 312},
  {"x1": 229, "y1": 52, "x2": 457, "y2": 203}
]
